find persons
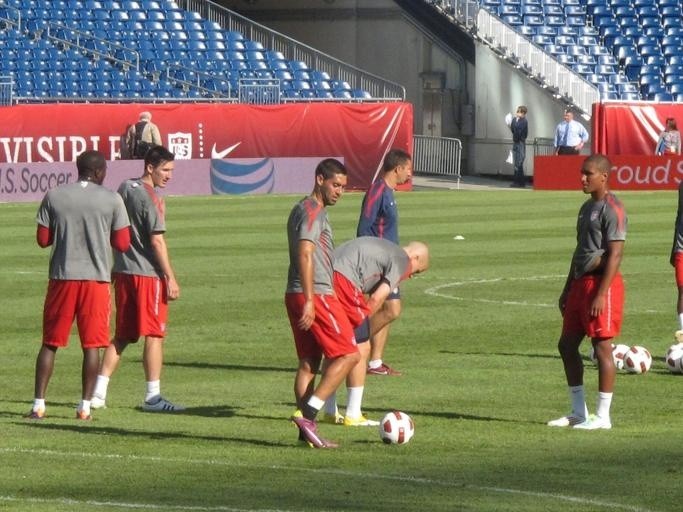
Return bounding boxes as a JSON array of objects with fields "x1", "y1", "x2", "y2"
[
  {"x1": 356, "y1": 148, "x2": 412, "y2": 376},
  {"x1": 285, "y1": 158, "x2": 361, "y2": 450},
  {"x1": 90, "y1": 145, "x2": 180, "y2": 412},
  {"x1": 507, "y1": 106, "x2": 528, "y2": 188},
  {"x1": 23, "y1": 150, "x2": 132, "y2": 421},
  {"x1": 125, "y1": 112, "x2": 163, "y2": 159},
  {"x1": 323, "y1": 236, "x2": 430, "y2": 427},
  {"x1": 670, "y1": 178, "x2": 683, "y2": 343},
  {"x1": 547, "y1": 154, "x2": 627, "y2": 431},
  {"x1": 553, "y1": 111, "x2": 589, "y2": 155},
  {"x1": 655, "y1": 118, "x2": 682, "y2": 156}
]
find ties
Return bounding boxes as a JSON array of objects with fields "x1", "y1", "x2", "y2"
[{"x1": 563, "y1": 123, "x2": 569, "y2": 146}]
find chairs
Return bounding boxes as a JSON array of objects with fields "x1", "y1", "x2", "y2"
[{"x1": 476, "y1": 0, "x2": 683, "y2": 101}]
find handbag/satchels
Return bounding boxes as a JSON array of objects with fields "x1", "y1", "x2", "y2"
[
  {"x1": 658, "y1": 139, "x2": 664, "y2": 152},
  {"x1": 137, "y1": 141, "x2": 155, "y2": 156}
]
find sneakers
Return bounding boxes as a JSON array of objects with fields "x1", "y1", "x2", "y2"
[
  {"x1": 367, "y1": 364, "x2": 401, "y2": 376},
  {"x1": 22, "y1": 409, "x2": 45, "y2": 419},
  {"x1": 291, "y1": 414, "x2": 382, "y2": 448},
  {"x1": 546, "y1": 412, "x2": 612, "y2": 428},
  {"x1": 143, "y1": 398, "x2": 184, "y2": 412},
  {"x1": 76, "y1": 409, "x2": 92, "y2": 420},
  {"x1": 510, "y1": 183, "x2": 524, "y2": 187},
  {"x1": 675, "y1": 331, "x2": 683, "y2": 343},
  {"x1": 91, "y1": 397, "x2": 107, "y2": 409}
]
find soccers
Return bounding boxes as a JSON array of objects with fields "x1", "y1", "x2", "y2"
[
  {"x1": 591, "y1": 343, "x2": 613, "y2": 368},
  {"x1": 612, "y1": 345, "x2": 630, "y2": 369},
  {"x1": 380, "y1": 411, "x2": 414, "y2": 445},
  {"x1": 623, "y1": 346, "x2": 651, "y2": 374},
  {"x1": 666, "y1": 343, "x2": 683, "y2": 373}
]
